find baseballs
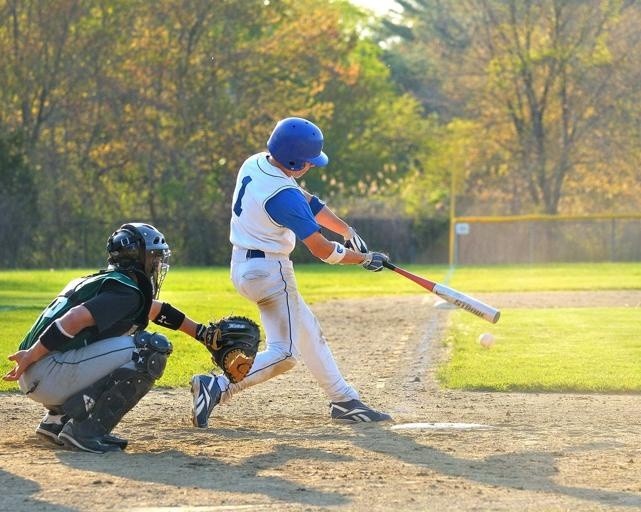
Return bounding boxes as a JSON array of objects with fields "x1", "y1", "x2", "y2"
[{"x1": 480, "y1": 333, "x2": 496, "y2": 349}]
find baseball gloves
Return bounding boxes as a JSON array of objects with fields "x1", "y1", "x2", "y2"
[{"x1": 204, "y1": 317, "x2": 260, "y2": 385}]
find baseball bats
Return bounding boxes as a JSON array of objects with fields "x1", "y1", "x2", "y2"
[{"x1": 383, "y1": 261, "x2": 500, "y2": 324}]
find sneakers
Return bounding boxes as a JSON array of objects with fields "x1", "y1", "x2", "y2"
[
  {"x1": 191, "y1": 375, "x2": 221, "y2": 428},
  {"x1": 58, "y1": 419, "x2": 128, "y2": 454},
  {"x1": 35, "y1": 411, "x2": 70, "y2": 446},
  {"x1": 331, "y1": 399, "x2": 391, "y2": 424}
]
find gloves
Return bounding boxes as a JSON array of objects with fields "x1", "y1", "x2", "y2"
[
  {"x1": 343, "y1": 228, "x2": 368, "y2": 254},
  {"x1": 359, "y1": 252, "x2": 389, "y2": 272}
]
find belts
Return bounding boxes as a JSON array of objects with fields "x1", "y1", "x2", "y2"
[{"x1": 246, "y1": 249, "x2": 264, "y2": 258}]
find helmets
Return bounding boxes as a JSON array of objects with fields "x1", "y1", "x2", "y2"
[
  {"x1": 267, "y1": 117, "x2": 329, "y2": 171},
  {"x1": 107, "y1": 223, "x2": 171, "y2": 300}
]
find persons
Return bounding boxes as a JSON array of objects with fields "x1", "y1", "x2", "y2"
[
  {"x1": 188, "y1": 114, "x2": 394, "y2": 428},
  {"x1": 3, "y1": 222, "x2": 264, "y2": 455}
]
